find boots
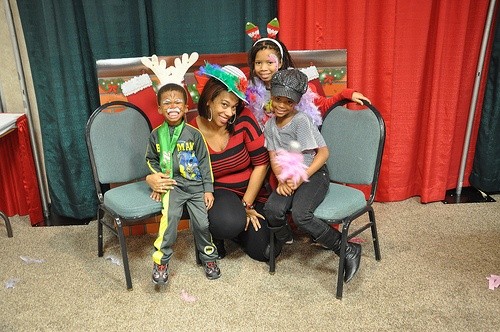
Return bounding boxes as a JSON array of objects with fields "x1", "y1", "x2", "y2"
[
  {"x1": 263, "y1": 217, "x2": 294, "y2": 260},
  {"x1": 312, "y1": 225, "x2": 363, "y2": 283}
]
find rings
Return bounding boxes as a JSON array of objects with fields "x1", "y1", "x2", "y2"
[{"x1": 162, "y1": 183, "x2": 164, "y2": 187}]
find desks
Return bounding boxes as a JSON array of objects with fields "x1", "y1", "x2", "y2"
[{"x1": 0, "y1": 113, "x2": 26, "y2": 238}]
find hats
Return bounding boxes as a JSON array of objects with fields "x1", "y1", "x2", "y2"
[
  {"x1": 269, "y1": 68, "x2": 309, "y2": 105},
  {"x1": 194, "y1": 63, "x2": 250, "y2": 105}
]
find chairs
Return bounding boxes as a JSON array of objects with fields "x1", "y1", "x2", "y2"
[
  {"x1": 268, "y1": 98, "x2": 386, "y2": 300},
  {"x1": 84, "y1": 101, "x2": 164, "y2": 291}
]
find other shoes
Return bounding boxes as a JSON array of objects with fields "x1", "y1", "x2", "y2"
[
  {"x1": 151, "y1": 263, "x2": 169, "y2": 285},
  {"x1": 204, "y1": 260, "x2": 222, "y2": 280}
]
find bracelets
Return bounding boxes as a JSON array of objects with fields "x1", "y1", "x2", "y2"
[{"x1": 241, "y1": 199, "x2": 255, "y2": 209}]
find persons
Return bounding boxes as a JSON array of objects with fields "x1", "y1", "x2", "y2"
[
  {"x1": 246, "y1": 39, "x2": 371, "y2": 250},
  {"x1": 146, "y1": 83, "x2": 221, "y2": 284},
  {"x1": 264, "y1": 69, "x2": 361, "y2": 283},
  {"x1": 146, "y1": 64, "x2": 282, "y2": 263}
]
